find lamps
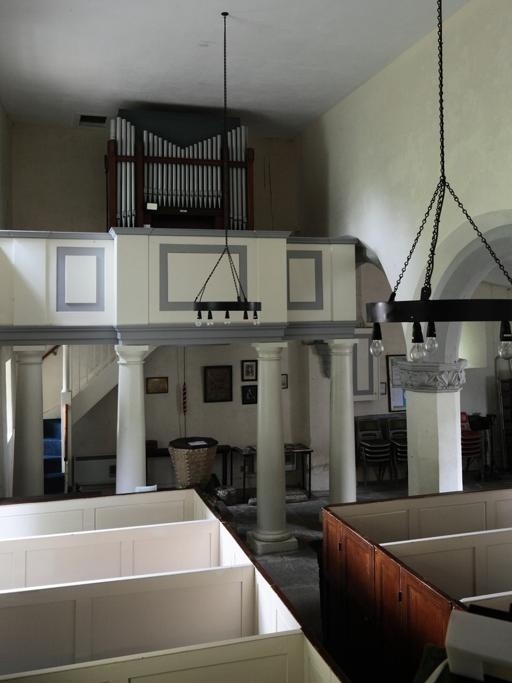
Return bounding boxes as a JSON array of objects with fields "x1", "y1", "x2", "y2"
[
  {"x1": 193, "y1": 12, "x2": 263, "y2": 326},
  {"x1": 363, "y1": 1, "x2": 512, "y2": 361}
]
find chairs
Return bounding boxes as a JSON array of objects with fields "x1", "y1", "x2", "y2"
[{"x1": 354, "y1": 411, "x2": 487, "y2": 489}]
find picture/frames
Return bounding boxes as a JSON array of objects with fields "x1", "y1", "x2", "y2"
[
  {"x1": 204, "y1": 365, "x2": 233, "y2": 402},
  {"x1": 240, "y1": 359, "x2": 258, "y2": 405},
  {"x1": 386, "y1": 354, "x2": 409, "y2": 413},
  {"x1": 281, "y1": 374, "x2": 289, "y2": 390},
  {"x1": 147, "y1": 377, "x2": 169, "y2": 393}
]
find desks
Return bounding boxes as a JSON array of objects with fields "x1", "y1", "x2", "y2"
[
  {"x1": 229, "y1": 444, "x2": 315, "y2": 503},
  {"x1": 146, "y1": 445, "x2": 230, "y2": 488}
]
